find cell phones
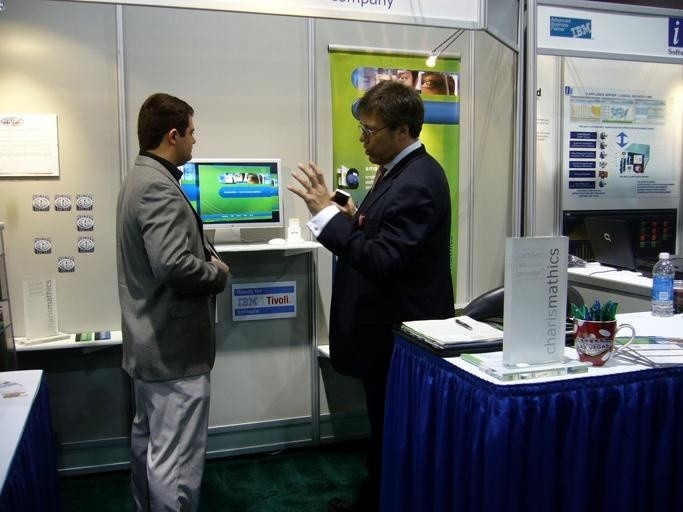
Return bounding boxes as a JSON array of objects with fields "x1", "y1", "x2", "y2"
[{"x1": 334, "y1": 189, "x2": 350, "y2": 206}]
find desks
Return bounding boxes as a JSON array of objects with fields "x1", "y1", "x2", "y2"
[{"x1": 381, "y1": 315, "x2": 682, "y2": 511}]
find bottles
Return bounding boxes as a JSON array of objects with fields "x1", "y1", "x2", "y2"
[{"x1": 652, "y1": 252, "x2": 675, "y2": 317}]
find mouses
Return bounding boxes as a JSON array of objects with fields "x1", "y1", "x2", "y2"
[{"x1": 268, "y1": 238, "x2": 286, "y2": 246}]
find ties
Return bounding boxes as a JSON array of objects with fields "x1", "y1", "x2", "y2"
[{"x1": 370, "y1": 168, "x2": 387, "y2": 194}]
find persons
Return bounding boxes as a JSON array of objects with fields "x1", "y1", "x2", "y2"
[
  {"x1": 355, "y1": 67, "x2": 455, "y2": 94},
  {"x1": 115, "y1": 92, "x2": 230, "y2": 512},
  {"x1": 286, "y1": 76, "x2": 456, "y2": 512}
]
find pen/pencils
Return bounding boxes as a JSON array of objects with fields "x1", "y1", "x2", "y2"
[
  {"x1": 456, "y1": 319, "x2": 472, "y2": 330},
  {"x1": 570, "y1": 300, "x2": 618, "y2": 321}
]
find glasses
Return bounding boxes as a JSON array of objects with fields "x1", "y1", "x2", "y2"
[{"x1": 360, "y1": 124, "x2": 390, "y2": 136}]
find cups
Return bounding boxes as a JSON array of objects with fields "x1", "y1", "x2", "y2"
[{"x1": 573, "y1": 319, "x2": 635, "y2": 368}]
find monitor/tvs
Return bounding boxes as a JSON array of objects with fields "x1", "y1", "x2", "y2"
[{"x1": 177, "y1": 158, "x2": 282, "y2": 246}]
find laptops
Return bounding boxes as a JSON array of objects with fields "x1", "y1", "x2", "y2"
[{"x1": 582, "y1": 214, "x2": 683, "y2": 280}]
